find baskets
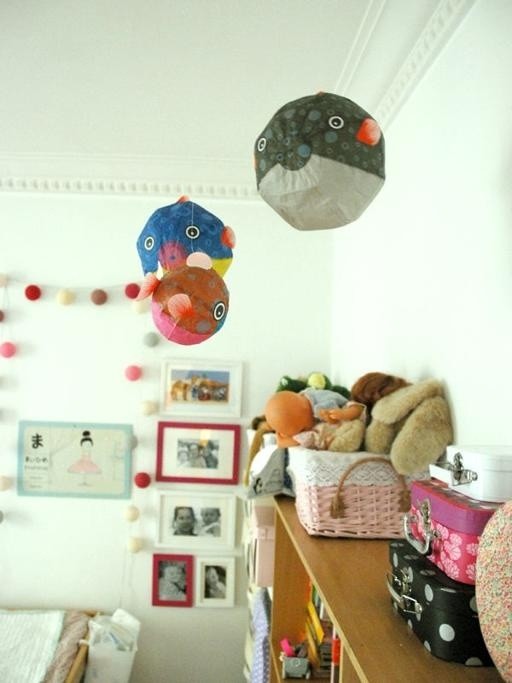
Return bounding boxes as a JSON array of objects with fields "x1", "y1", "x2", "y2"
[{"x1": 286, "y1": 445, "x2": 413, "y2": 540}]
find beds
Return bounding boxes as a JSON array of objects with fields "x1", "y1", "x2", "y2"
[{"x1": 0, "y1": 609, "x2": 102, "y2": 683}]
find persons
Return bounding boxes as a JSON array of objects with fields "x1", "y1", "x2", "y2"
[
  {"x1": 158, "y1": 562, "x2": 186, "y2": 603},
  {"x1": 171, "y1": 506, "x2": 222, "y2": 537},
  {"x1": 177, "y1": 441, "x2": 221, "y2": 469},
  {"x1": 205, "y1": 568, "x2": 226, "y2": 598}
]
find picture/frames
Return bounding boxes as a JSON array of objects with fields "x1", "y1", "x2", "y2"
[
  {"x1": 161, "y1": 358, "x2": 242, "y2": 416},
  {"x1": 152, "y1": 553, "x2": 193, "y2": 607},
  {"x1": 195, "y1": 556, "x2": 235, "y2": 608},
  {"x1": 156, "y1": 421, "x2": 241, "y2": 485},
  {"x1": 16, "y1": 420, "x2": 133, "y2": 500},
  {"x1": 156, "y1": 488, "x2": 236, "y2": 551}
]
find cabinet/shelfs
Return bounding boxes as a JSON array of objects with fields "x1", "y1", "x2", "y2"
[{"x1": 266, "y1": 498, "x2": 508, "y2": 683}]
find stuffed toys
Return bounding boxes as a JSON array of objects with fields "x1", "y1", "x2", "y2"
[{"x1": 243, "y1": 372, "x2": 455, "y2": 490}]
